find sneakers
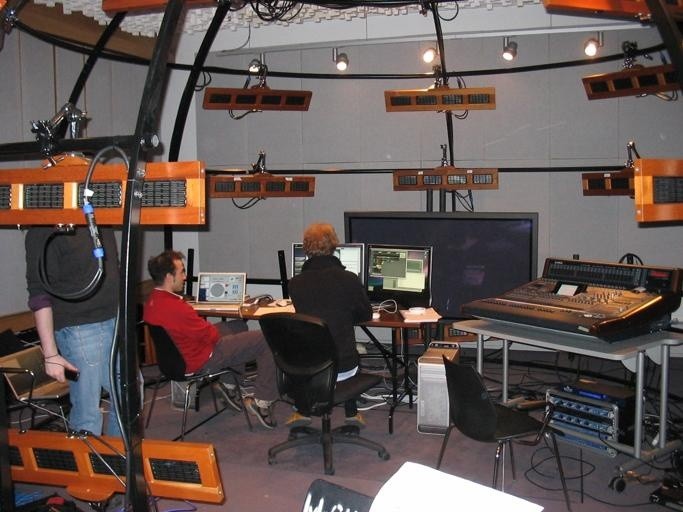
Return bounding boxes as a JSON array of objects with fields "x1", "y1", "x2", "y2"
[
  {"x1": 243, "y1": 397, "x2": 277, "y2": 429},
  {"x1": 286, "y1": 413, "x2": 311, "y2": 427},
  {"x1": 346, "y1": 414, "x2": 366, "y2": 428},
  {"x1": 216, "y1": 382, "x2": 243, "y2": 412}
]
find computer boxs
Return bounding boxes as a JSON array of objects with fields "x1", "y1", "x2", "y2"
[{"x1": 417, "y1": 342, "x2": 460, "y2": 436}]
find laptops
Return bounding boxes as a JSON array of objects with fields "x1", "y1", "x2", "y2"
[{"x1": 191, "y1": 272, "x2": 247, "y2": 311}]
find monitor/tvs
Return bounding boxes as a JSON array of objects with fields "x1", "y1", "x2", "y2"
[
  {"x1": 291, "y1": 242, "x2": 364, "y2": 286},
  {"x1": 344, "y1": 211, "x2": 538, "y2": 319},
  {"x1": 365, "y1": 243, "x2": 433, "y2": 308}
]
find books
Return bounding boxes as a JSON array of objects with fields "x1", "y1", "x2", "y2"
[{"x1": 399, "y1": 309, "x2": 442, "y2": 322}]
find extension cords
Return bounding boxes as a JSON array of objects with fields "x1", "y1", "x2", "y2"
[{"x1": 516, "y1": 400, "x2": 546, "y2": 410}]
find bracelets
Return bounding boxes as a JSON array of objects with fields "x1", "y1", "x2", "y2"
[{"x1": 44, "y1": 353, "x2": 58, "y2": 360}]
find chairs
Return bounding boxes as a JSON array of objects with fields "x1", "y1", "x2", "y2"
[
  {"x1": 137, "y1": 316, "x2": 256, "y2": 444},
  {"x1": 0, "y1": 342, "x2": 71, "y2": 436},
  {"x1": 252, "y1": 306, "x2": 394, "y2": 477},
  {"x1": 431, "y1": 351, "x2": 577, "y2": 512}
]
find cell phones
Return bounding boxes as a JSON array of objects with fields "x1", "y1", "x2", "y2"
[{"x1": 64, "y1": 369, "x2": 80, "y2": 381}]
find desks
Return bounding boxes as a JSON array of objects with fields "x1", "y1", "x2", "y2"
[
  {"x1": 172, "y1": 297, "x2": 441, "y2": 436},
  {"x1": 444, "y1": 313, "x2": 682, "y2": 464}
]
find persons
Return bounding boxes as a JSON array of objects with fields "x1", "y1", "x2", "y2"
[
  {"x1": 286, "y1": 224, "x2": 373, "y2": 431},
  {"x1": 25, "y1": 155, "x2": 144, "y2": 437},
  {"x1": 144, "y1": 251, "x2": 279, "y2": 430}
]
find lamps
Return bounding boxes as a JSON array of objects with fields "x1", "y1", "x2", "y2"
[
  {"x1": 422, "y1": 47, "x2": 437, "y2": 63},
  {"x1": 337, "y1": 53, "x2": 349, "y2": 70},
  {"x1": 585, "y1": 38, "x2": 599, "y2": 56},
  {"x1": 503, "y1": 41, "x2": 518, "y2": 61},
  {"x1": 248, "y1": 59, "x2": 260, "y2": 73}
]
